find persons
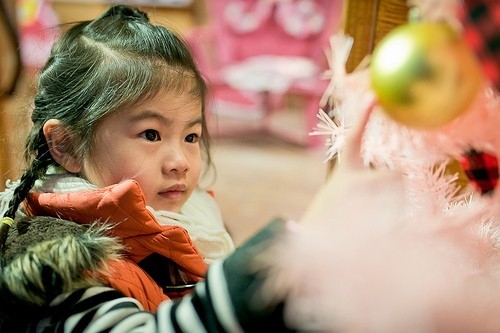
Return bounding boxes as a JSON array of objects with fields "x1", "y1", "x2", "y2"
[{"x1": 0, "y1": 4, "x2": 290, "y2": 333}]
